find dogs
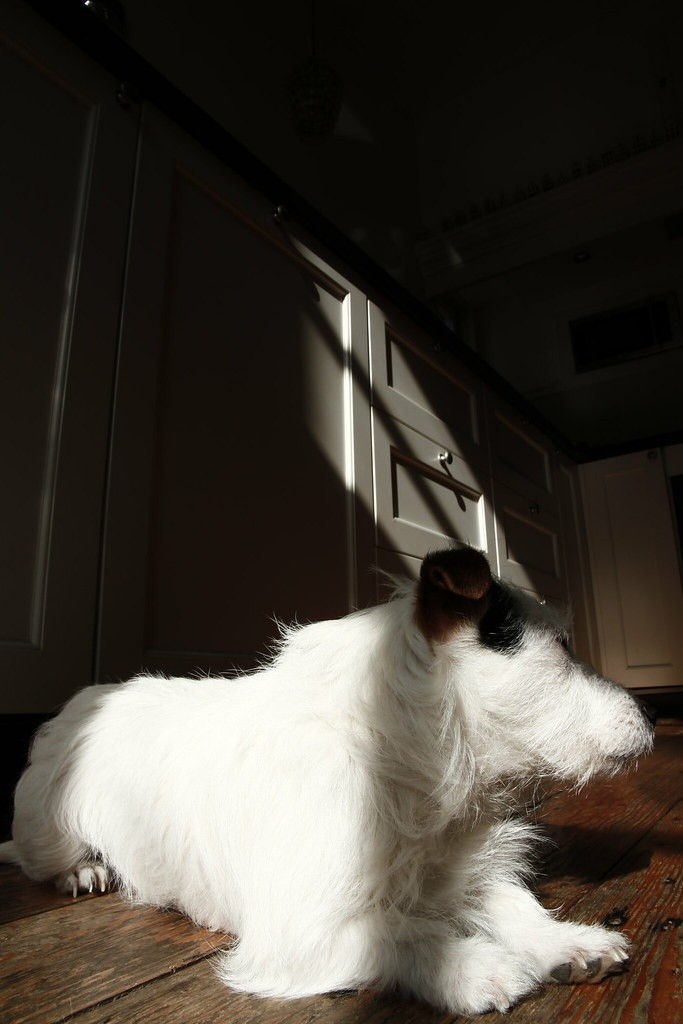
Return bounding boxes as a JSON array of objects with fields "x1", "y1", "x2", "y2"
[{"x1": 1, "y1": 544, "x2": 655, "y2": 1015}]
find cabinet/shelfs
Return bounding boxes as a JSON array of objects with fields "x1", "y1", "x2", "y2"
[
  {"x1": 576, "y1": 444, "x2": 683, "y2": 692},
  {"x1": 0, "y1": 0, "x2": 143, "y2": 715},
  {"x1": 98, "y1": 96, "x2": 608, "y2": 680}
]
